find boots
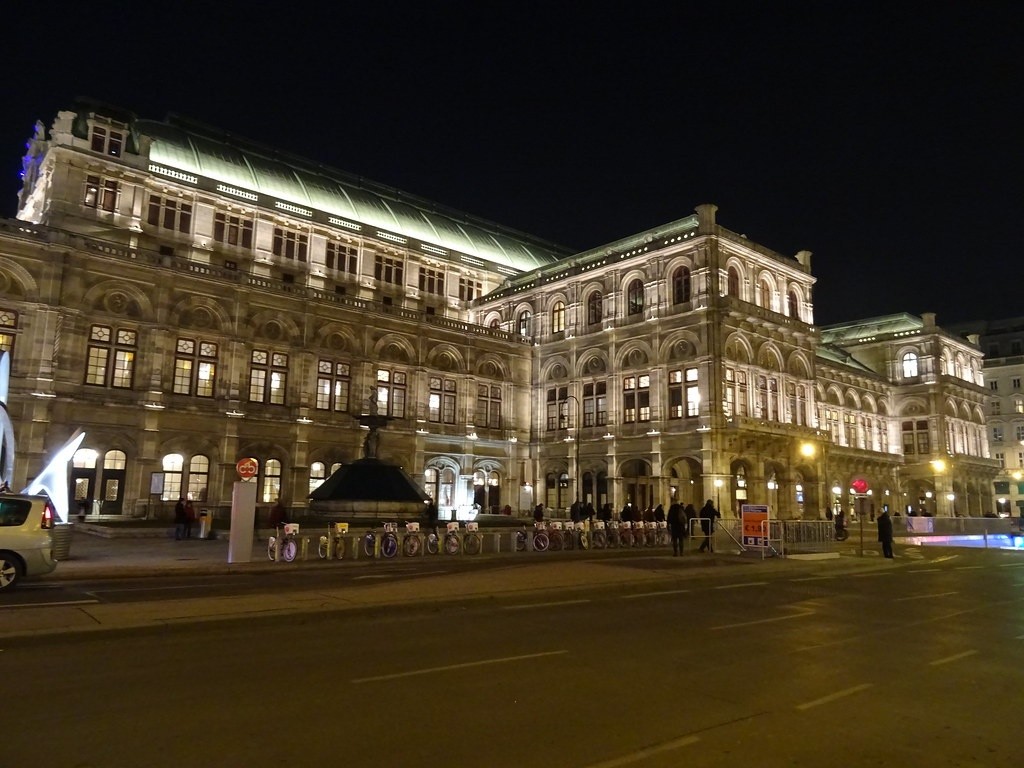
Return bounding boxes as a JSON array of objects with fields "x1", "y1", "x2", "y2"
[
  {"x1": 673, "y1": 544, "x2": 678, "y2": 556},
  {"x1": 680, "y1": 544, "x2": 684, "y2": 556}
]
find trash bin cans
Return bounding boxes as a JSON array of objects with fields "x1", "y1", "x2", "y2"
[{"x1": 198, "y1": 509, "x2": 212, "y2": 539}]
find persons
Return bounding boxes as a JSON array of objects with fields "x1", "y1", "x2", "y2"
[
  {"x1": 877, "y1": 511, "x2": 894, "y2": 558},
  {"x1": 424, "y1": 498, "x2": 441, "y2": 541},
  {"x1": 175, "y1": 497, "x2": 195, "y2": 541},
  {"x1": 570, "y1": 501, "x2": 596, "y2": 524},
  {"x1": 369, "y1": 386, "x2": 378, "y2": 405},
  {"x1": 603, "y1": 502, "x2": 665, "y2": 528},
  {"x1": 534, "y1": 503, "x2": 544, "y2": 522},
  {"x1": 825, "y1": 507, "x2": 833, "y2": 521},
  {"x1": 699, "y1": 499, "x2": 721, "y2": 553},
  {"x1": 666, "y1": 501, "x2": 698, "y2": 557}
]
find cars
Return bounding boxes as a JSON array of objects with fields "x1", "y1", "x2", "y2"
[{"x1": 0, "y1": 493, "x2": 59, "y2": 592}]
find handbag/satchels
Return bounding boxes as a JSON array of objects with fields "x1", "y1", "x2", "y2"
[{"x1": 678, "y1": 509, "x2": 687, "y2": 525}]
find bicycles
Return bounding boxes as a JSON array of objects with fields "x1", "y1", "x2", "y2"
[
  {"x1": 267, "y1": 521, "x2": 300, "y2": 563},
  {"x1": 402, "y1": 520, "x2": 422, "y2": 557},
  {"x1": 318, "y1": 521, "x2": 353, "y2": 560},
  {"x1": 444, "y1": 521, "x2": 460, "y2": 556},
  {"x1": 548, "y1": 518, "x2": 671, "y2": 551},
  {"x1": 364, "y1": 520, "x2": 399, "y2": 558},
  {"x1": 462, "y1": 520, "x2": 482, "y2": 555},
  {"x1": 516, "y1": 519, "x2": 550, "y2": 551},
  {"x1": 424, "y1": 520, "x2": 440, "y2": 555}
]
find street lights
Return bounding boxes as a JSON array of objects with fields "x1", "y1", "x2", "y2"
[
  {"x1": 801, "y1": 432, "x2": 830, "y2": 522},
  {"x1": 932, "y1": 454, "x2": 953, "y2": 518}
]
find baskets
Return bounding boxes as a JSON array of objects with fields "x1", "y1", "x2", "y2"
[
  {"x1": 535, "y1": 521, "x2": 668, "y2": 530},
  {"x1": 282, "y1": 520, "x2": 479, "y2": 535}
]
